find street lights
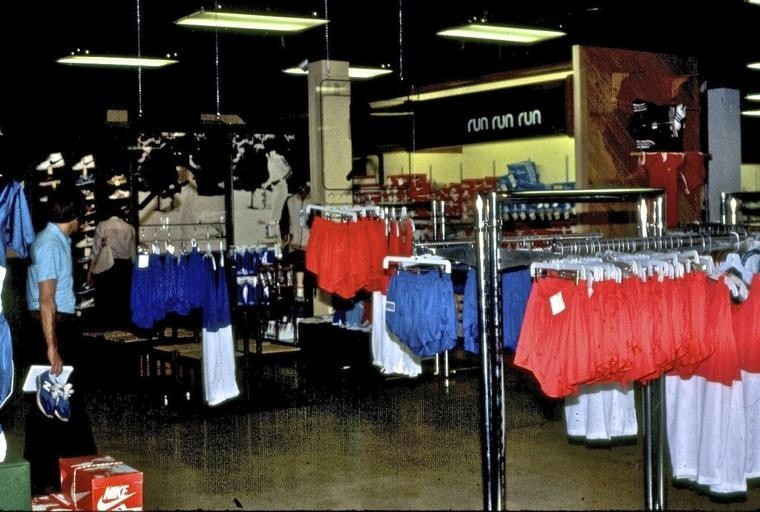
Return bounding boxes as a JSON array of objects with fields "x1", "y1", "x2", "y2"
[{"x1": 92, "y1": 222, "x2": 114, "y2": 274}]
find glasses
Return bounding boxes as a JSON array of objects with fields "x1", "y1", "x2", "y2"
[{"x1": 174, "y1": 51, "x2": 180, "y2": 57}]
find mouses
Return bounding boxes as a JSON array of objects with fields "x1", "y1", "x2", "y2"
[
  {"x1": 413, "y1": 184, "x2": 752, "y2": 510},
  {"x1": 398, "y1": 252, "x2": 446, "y2": 268},
  {"x1": 298, "y1": 201, "x2": 451, "y2": 382},
  {"x1": 390, "y1": 207, "x2": 400, "y2": 238},
  {"x1": 151, "y1": 226, "x2": 161, "y2": 255},
  {"x1": 219, "y1": 223, "x2": 224, "y2": 266},
  {"x1": 325, "y1": 192, "x2": 367, "y2": 221},
  {"x1": 530, "y1": 234, "x2": 726, "y2": 287},
  {"x1": 382, "y1": 255, "x2": 452, "y2": 275},
  {"x1": 191, "y1": 226, "x2": 200, "y2": 254},
  {"x1": 202, "y1": 225, "x2": 217, "y2": 270},
  {"x1": 384, "y1": 208, "x2": 389, "y2": 238},
  {"x1": 165, "y1": 225, "x2": 175, "y2": 255},
  {"x1": 137, "y1": 222, "x2": 226, "y2": 227},
  {"x1": 364, "y1": 204, "x2": 382, "y2": 218},
  {"x1": 718, "y1": 231, "x2": 752, "y2": 285},
  {"x1": 138, "y1": 227, "x2": 150, "y2": 255},
  {"x1": 400, "y1": 206, "x2": 416, "y2": 233},
  {"x1": 180, "y1": 226, "x2": 188, "y2": 258},
  {"x1": 305, "y1": 193, "x2": 357, "y2": 225}
]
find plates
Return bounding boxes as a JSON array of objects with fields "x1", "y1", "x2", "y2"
[{"x1": 30, "y1": 144, "x2": 136, "y2": 326}]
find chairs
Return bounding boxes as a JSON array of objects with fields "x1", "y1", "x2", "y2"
[
  {"x1": 39, "y1": 176, "x2": 61, "y2": 186},
  {"x1": 35, "y1": 368, "x2": 61, "y2": 420},
  {"x1": 82, "y1": 190, "x2": 94, "y2": 200},
  {"x1": 36, "y1": 152, "x2": 65, "y2": 171},
  {"x1": 109, "y1": 189, "x2": 130, "y2": 201},
  {"x1": 107, "y1": 173, "x2": 127, "y2": 186},
  {"x1": 40, "y1": 196, "x2": 48, "y2": 203},
  {"x1": 85, "y1": 204, "x2": 95, "y2": 216},
  {"x1": 75, "y1": 297, "x2": 95, "y2": 311},
  {"x1": 71, "y1": 154, "x2": 95, "y2": 171},
  {"x1": 74, "y1": 174, "x2": 95, "y2": 185},
  {"x1": 54, "y1": 383, "x2": 74, "y2": 423},
  {"x1": 76, "y1": 256, "x2": 90, "y2": 263},
  {"x1": 80, "y1": 220, "x2": 96, "y2": 231},
  {"x1": 76, "y1": 236, "x2": 94, "y2": 248},
  {"x1": 75, "y1": 282, "x2": 96, "y2": 294}
]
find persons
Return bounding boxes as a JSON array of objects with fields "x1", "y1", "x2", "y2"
[
  {"x1": 24, "y1": 185, "x2": 96, "y2": 496},
  {"x1": 87, "y1": 204, "x2": 135, "y2": 346}
]
